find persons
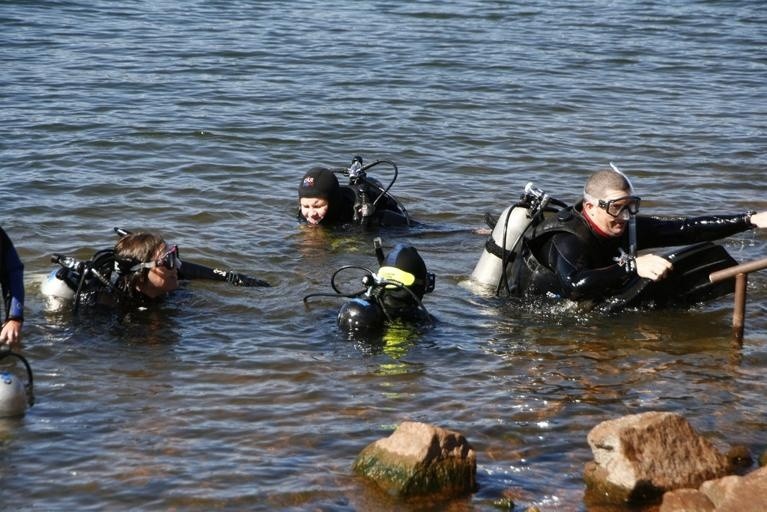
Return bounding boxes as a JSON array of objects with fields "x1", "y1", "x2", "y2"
[
  {"x1": 39, "y1": 226, "x2": 270, "y2": 322},
  {"x1": 1, "y1": 367, "x2": 36, "y2": 420},
  {"x1": 297, "y1": 166, "x2": 410, "y2": 252},
  {"x1": 517, "y1": 169, "x2": 767, "y2": 310},
  {"x1": 340, "y1": 245, "x2": 439, "y2": 339},
  {"x1": 1, "y1": 223, "x2": 25, "y2": 354}
]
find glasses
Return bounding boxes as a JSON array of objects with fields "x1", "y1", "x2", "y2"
[
  {"x1": 415, "y1": 273, "x2": 434, "y2": 293},
  {"x1": 583, "y1": 186, "x2": 642, "y2": 218},
  {"x1": 130, "y1": 244, "x2": 181, "y2": 273}
]
